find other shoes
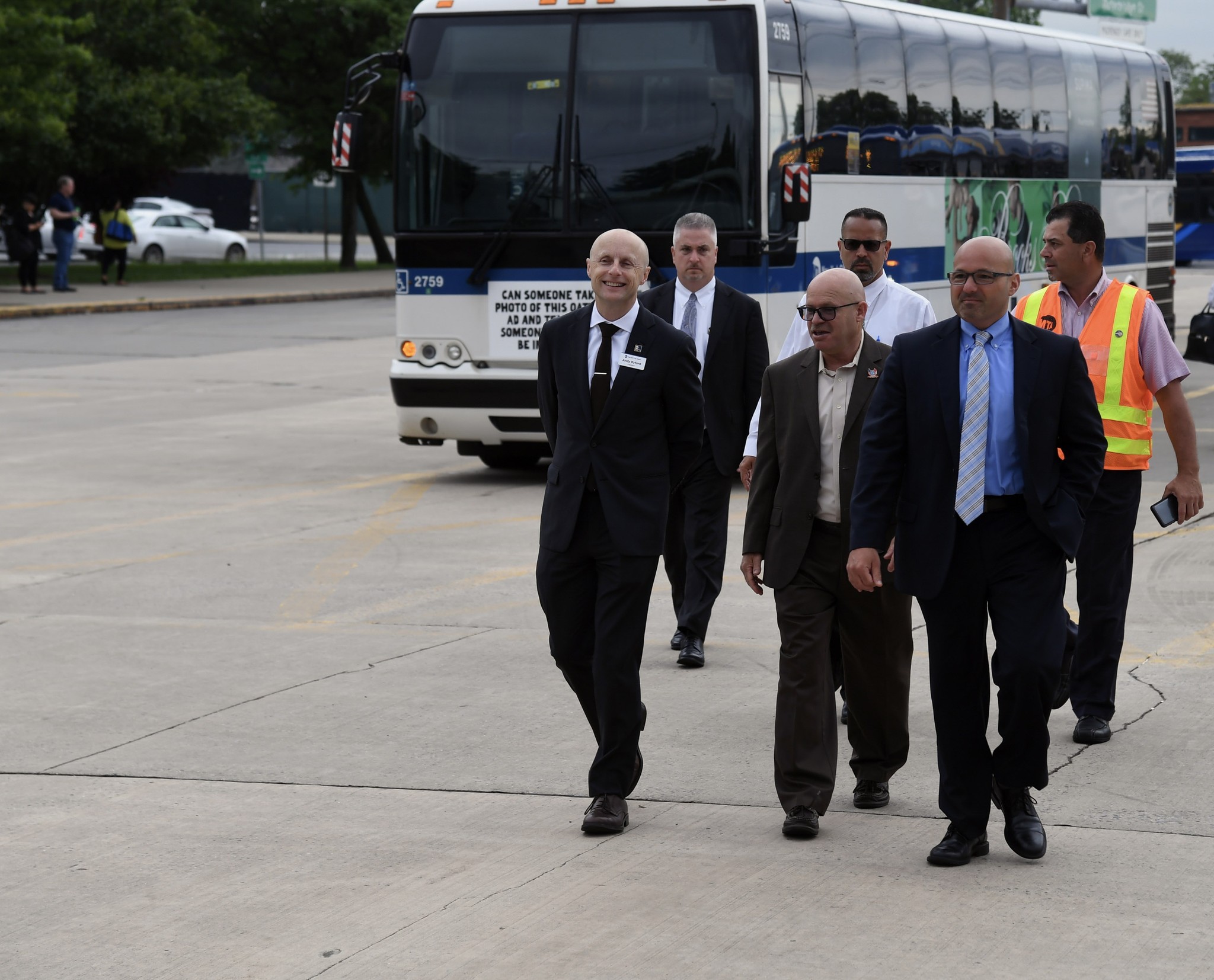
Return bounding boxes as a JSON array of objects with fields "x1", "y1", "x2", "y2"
[
  {"x1": 54, "y1": 286, "x2": 76, "y2": 292},
  {"x1": 20, "y1": 289, "x2": 32, "y2": 294},
  {"x1": 116, "y1": 279, "x2": 127, "y2": 286},
  {"x1": 101, "y1": 274, "x2": 108, "y2": 285},
  {"x1": 32, "y1": 289, "x2": 46, "y2": 294}
]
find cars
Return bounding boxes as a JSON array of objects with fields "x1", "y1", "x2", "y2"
[{"x1": 0, "y1": 195, "x2": 250, "y2": 266}]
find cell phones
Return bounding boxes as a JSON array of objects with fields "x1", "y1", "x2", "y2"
[{"x1": 1150, "y1": 493, "x2": 1179, "y2": 528}]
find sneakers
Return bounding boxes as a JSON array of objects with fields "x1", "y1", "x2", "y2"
[
  {"x1": 852, "y1": 778, "x2": 890, "y2": 809},
  {"x1": 781, "y1": 805, "x2": 820, "y2": 837}
]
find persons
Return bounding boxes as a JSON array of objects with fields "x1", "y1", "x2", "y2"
[
  {"x1": 740, "y1": 268, "x2": 916, "y2": 842},
  {"x1": 739, "y1": 209, "x2": 939, "y2": 724},
  {"x1": 534, "y1": 228, "x2": 707, "y2": 838},
  {"x1": 18, "y1": 198, "x2": 46, "y2": 294},
  {"x1": 1012, "y1": 201, "x2": 1205, "y2": 743},
  {"x1": 47, "y1": 176, "x2": 79, "y2": 293},
  {"x1": 637, "y1": 213, "x2": 770, "y2": 668},
  {"x1": 846, "y1": 236, "x2": 1108, "y2": 868},
  {"x1": 99, "y1": 195, "x2": 137, "y2": 287}
]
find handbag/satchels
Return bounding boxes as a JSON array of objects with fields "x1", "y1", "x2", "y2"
[
  {"x1": 106, "y1": 219, "x2": 133, "y2": 243},
  {"x1": 1182, "y1": 302, "x2": 1214, "y2": 366}
]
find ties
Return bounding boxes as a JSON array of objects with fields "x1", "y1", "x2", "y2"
[
  {"x1": 954, "y1": 329, "x2": 993, "y2": 527},
  {"x1": 589, "y1": 321, "x2": 621, "y2": 433},
  {"x1": 679, "y1": 293, "x2": 697, "y2": 343}
]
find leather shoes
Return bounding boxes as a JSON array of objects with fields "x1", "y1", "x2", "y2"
[
  {"x1": 1051, "y1": 646, "x2": 1074, "y2": 710},
  {"x1": 991, "y1": 774, "x2": 1047, "y2": 860},
  {"x1": 676, "y1": 634, "x2": 706, "y2": 667},
  {"x1": 841, "y1": 699, "x2": 847, "y2": 724},
  {"x1": 624, "y1": 702, "x2": 647, "y2": 800},
  {"x1": 580, "y1": 794, "x2": 630, "y2": 831},
  {"x1": 1072, "y1": 714, "x2": 1111, "y2": 745},
  {"x1": 926, "y1": 820, "x2": 990, "y2": 867},
  {"x1": 670, "y1": 628, "x2": 685, "y2": 650}
]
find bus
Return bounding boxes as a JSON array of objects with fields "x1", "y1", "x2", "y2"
[{"x1": 326, "y1": 1, "x2": 1180, "y2": 473}]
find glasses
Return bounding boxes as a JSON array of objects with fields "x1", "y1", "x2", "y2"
[
  {"x1": 947, "y1": 270, "x2": 1014, "y2": 286},
  {"x1": 796, "y1": 302, "x2": 859, "y2": 321},
  {"x1": 840, "y1": 237, "x2": 887, "y2": 251}
]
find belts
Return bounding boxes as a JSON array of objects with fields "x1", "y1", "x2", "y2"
[
  {"x1": 813, "y1": 519, "x2": 842, "y2": 541},
  {"x1": 983, "y1": 497, "x2": 1024, "y2": 513}
]
